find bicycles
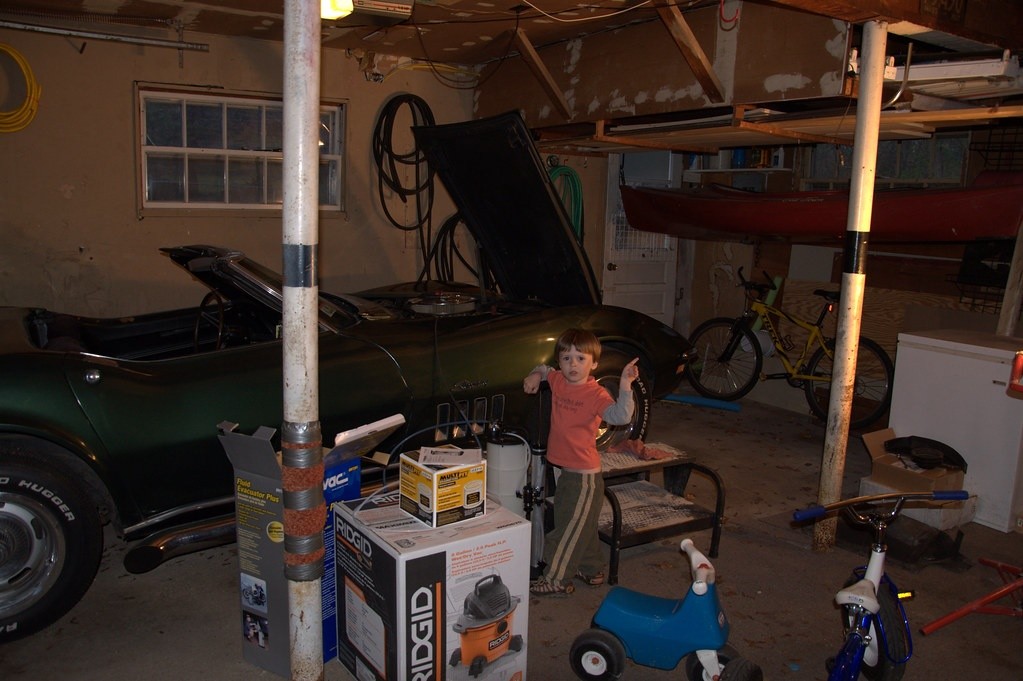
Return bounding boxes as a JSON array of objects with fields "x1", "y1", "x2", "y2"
[
  {"x1": 682, "y1": 266, "x2": 894, "y2": 429},
  {"x1": 790, "y1": 490, "x2": 972, "y2": 681}
]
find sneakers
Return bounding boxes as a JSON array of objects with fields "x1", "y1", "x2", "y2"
[
  {"x1": 530, "y1": 581, "x2": 575, "y2": 597},
  {"x1": 575, "y1": 569, "x2": 604, "y2": 587}
]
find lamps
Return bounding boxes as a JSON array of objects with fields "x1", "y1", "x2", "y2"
[{"x1": 320, "y1": 0, "x2": 354, "y2": 20}]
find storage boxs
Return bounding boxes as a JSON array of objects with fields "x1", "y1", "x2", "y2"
[
  {"x1": 399, "y1": 445, "x2": 486, "y2": 528},
  {"x1": 334, "y1": 491, "x2": 531, "y2": 681},
  {"x1": 862, "y1": 428, "x2": 965, "y2": 503},
  {"x1": 216, "y1": 414, "x2": 406, "y2": 680}
]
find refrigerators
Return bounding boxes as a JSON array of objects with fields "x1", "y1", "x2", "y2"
[{"x1": 888, "y1": 329, "x2": 1023, "y2": 532}]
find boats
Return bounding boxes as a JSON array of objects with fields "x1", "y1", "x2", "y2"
[{"x1": 616, "y1": 177, "x2": 1023, "y2": 250}]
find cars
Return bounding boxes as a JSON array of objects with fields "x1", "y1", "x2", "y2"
[{"x1": 1, "y1": 242, "x2": 689, "y2": 647}]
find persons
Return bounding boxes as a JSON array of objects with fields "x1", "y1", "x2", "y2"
[{"x1": 521, "y1": 327, "x2": 640, "y2": 595}]
